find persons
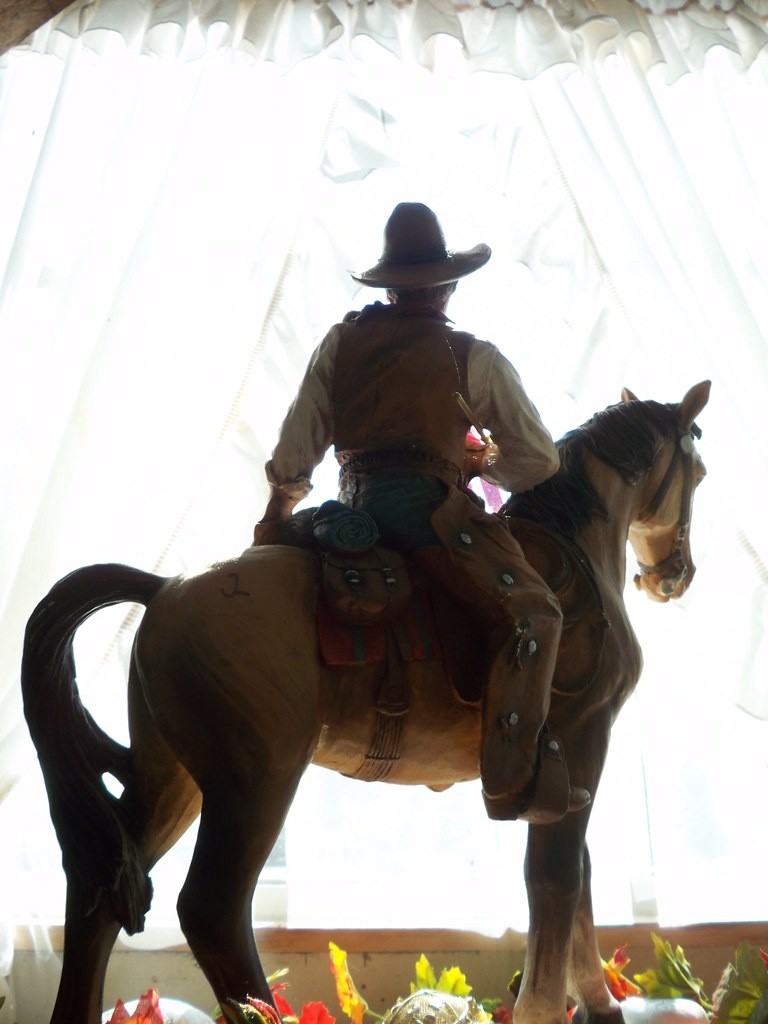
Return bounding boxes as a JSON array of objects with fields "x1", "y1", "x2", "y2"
[{"x1": 254, "y1": 202, "x2": 591, "y2": 823}]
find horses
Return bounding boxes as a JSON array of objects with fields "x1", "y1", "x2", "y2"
[{"x1": 21, "y1": 378, "x2": 712, "y2": 1024}]
